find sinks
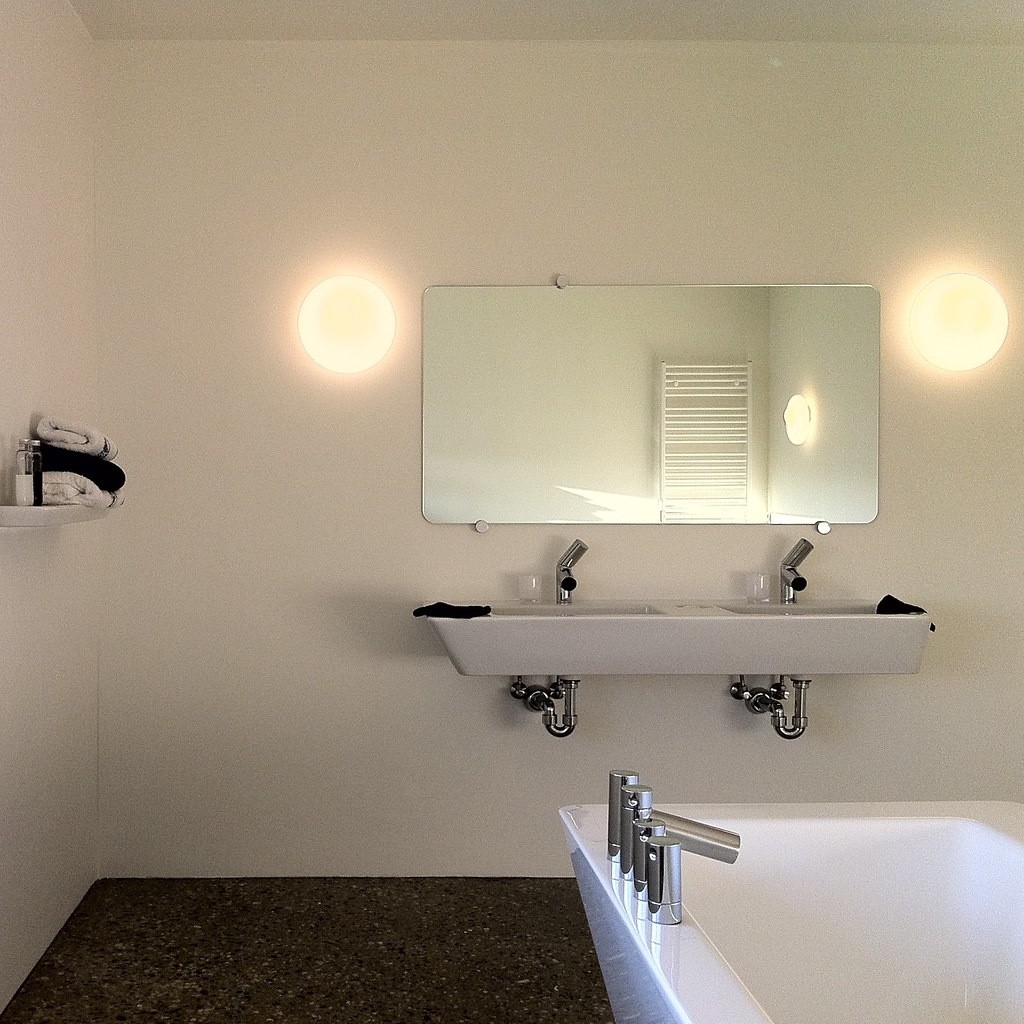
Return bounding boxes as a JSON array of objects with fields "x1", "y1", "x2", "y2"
[
  {"x1": 425, "y1": 594, "x2": 679, "y2": 682},
  {"x1": 696, "y1": 591, "x2": 933, "y2": 677}
]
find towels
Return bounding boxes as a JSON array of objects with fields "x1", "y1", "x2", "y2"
[
  {"x1": 875, "y1": 594, "x2": 938, "y2": 634},
  {"x1": 35, "y1": 416, "x2": 120, "y2": 463},
  {"x1": 28, "y1": 470, "x2": 126, "y2": 509},
  {"x1": 412, "y1": 600, "x2": 494, "y2": 621},
  {"x1": 28, "y1": 441, "x2": 126, "y2": 493}
]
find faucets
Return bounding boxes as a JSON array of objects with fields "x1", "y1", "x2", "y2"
[
  {"x1": 552, "y1": 538, "x2": 589, "y2": 606},
  {"x1": 606, "y1": 769, "x2": 743, "y2": 870},
  {"x1": 779, "y1": 537, "x2": 814, "y2": 605}
]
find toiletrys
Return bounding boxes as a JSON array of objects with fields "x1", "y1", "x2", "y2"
[
  {"x1": 26, "y1": 439, "x2": 44, "y2": 506},
  {"x1": 15, "y1": 437, "x2": 37, "y2": 507}
]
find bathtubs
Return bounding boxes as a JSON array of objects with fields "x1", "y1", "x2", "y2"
[{"x1": 559, "y1": 788, "x2": 1023, "y2": 1024}]
